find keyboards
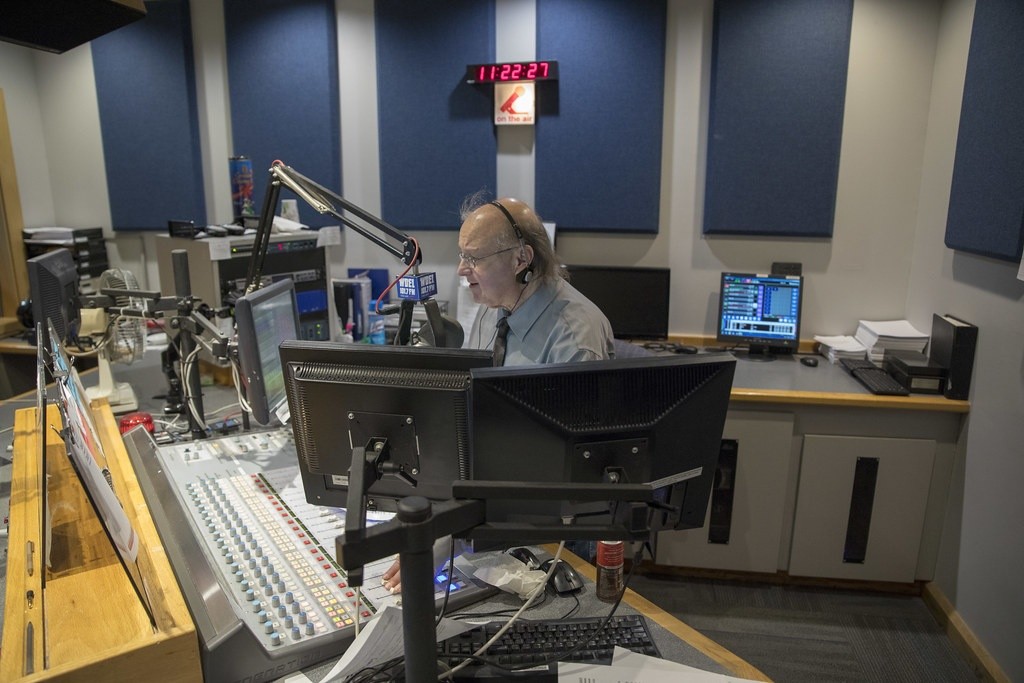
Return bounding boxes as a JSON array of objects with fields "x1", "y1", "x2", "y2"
[
  {"x1": 838, "y1": 358, "x2": 910, "y2": 396},
  {"x1": 373, "y1": 614, "x2": 664, "y2": 683}
]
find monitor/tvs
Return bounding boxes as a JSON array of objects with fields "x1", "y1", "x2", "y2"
[
  {"x1": 277, "y1": 337, "x2": 737, "y2": 683},
  {"x1": 26, "y1": 248, "x2": 82, "y2": 364},
  {"x1": 717, "y1": 273, "x2": 803, "y2": 363},
  {"x1": 170, "y1": 278, "x2": 303, "y2": 440},
  {"x1": 559, "y1": 264, "x2": 671, "y2": 340}
]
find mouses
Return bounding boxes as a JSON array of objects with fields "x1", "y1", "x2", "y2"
[
  {"x1": 507, "y1": 548, "x2": 540, "y2": 571},
  {"x1": 674, "y1": 346, "x2": 697, "y2": 354},
  {"x1": 800, "y1": 357, "x2": 818, "y2": 367},
  {"x1": 540, "y1": 557, "x2": 584, "y2": 594}
]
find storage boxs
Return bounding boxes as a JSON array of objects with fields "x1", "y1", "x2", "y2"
[{"x1": 883, "y1": 349, "x2": 948, "y2": 395}]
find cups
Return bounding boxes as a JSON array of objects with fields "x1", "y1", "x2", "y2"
[{"x1": 281, "y1": 199, "x2": 300, "y2": 232}]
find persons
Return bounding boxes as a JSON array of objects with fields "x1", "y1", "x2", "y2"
[{"x1": 381, "y1": 197, "x2": 616, "y2": 596}]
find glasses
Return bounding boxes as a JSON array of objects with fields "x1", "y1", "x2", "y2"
[{"x1": 458, "y1": 245, "x2": 523, "y2": 268}]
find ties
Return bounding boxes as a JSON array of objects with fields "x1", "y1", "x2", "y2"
[{"x1": 491, "y1": 316, "x2": 509, "y2": 368}]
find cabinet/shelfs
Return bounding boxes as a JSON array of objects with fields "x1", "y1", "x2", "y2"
[
  {"x1": 22, "y1": 227, "x2": 109, "y2": 280},
  {"x1": 621, "y1": 349, "x2": 974, "y2": 596}
]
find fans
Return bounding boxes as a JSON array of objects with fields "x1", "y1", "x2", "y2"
[{"x1": 86, "y1": 266, "x2": 145, "y2": 416}]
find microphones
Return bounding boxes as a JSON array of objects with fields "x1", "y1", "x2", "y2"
[{"x1": 406, "y1": 314, "x2": 465, "y2": 348}]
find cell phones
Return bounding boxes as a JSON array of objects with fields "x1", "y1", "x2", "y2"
[{"x1": 207, "y1": 418, "x2": 241, "y2": 431}]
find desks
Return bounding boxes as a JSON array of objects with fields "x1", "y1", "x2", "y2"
[{"x1": 0, "y1": 335, "x2": 133, "y2": 402}]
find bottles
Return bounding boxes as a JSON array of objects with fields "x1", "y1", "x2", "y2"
[
  {"x1": 369, "y1": 300, "x2": 384, "y2": 343},
  {"x1": 596, "y1": 541, "x2": 623, "y2": 603}
]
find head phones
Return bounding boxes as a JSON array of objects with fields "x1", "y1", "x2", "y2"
[{"x1": 491, "y1": 201, "x2": 534, "y2": 284}]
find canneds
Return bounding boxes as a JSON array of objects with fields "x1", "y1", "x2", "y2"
[
  {"x1": 369, "y1": 300, "x2": 386, "y2": 344},
  {"x1": 596, "y1": 542, "x2": 625, "y2": 603}
]
